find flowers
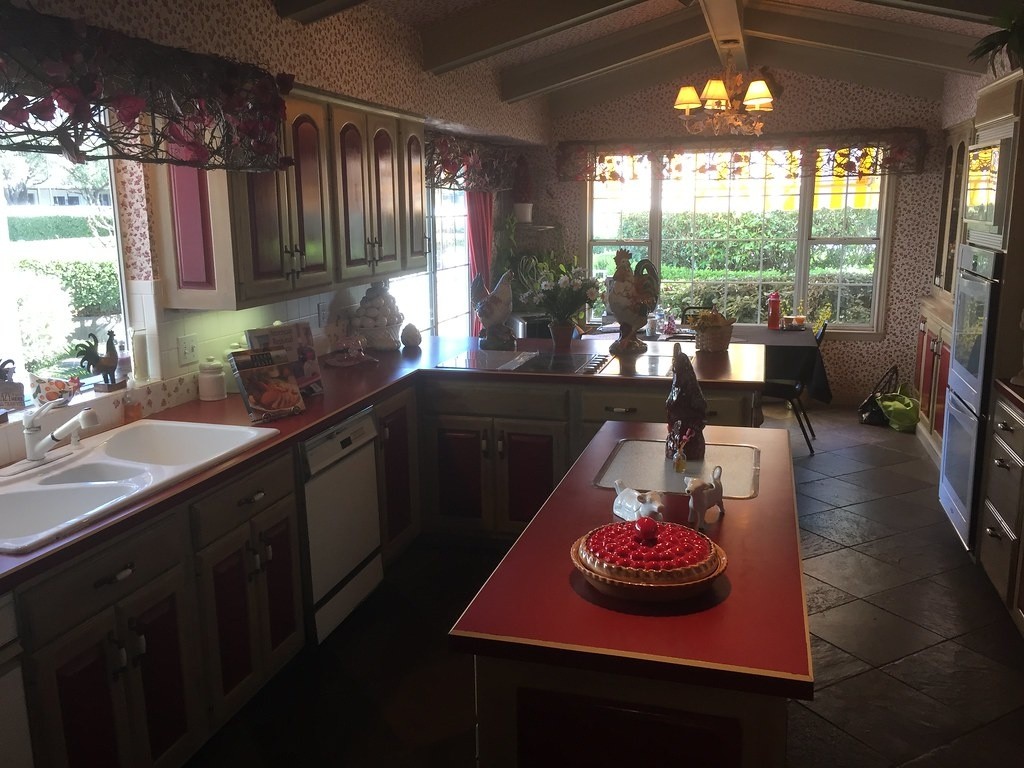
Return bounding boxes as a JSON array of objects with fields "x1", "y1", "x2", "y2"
[
  {"x1": 685, "y1": 306, "x2": 738, "y2": 331},
  {"x1": 548, "y1": 321, "x2": 576, "y2": 349},
  {"x1": 519, "y1": 264, "x2": 600, "y2": 324}
]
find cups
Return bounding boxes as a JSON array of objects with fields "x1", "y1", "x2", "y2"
[
  {"x1": 783, "y1": 316, "x2": 794, "y2": 330},
  {"x1": 794, "y1": 315, "x2": 806, "y2": 330}
]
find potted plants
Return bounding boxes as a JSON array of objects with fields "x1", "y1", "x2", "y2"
[{"x1": 511, "y1": 171, "x2": 533, "y2": 225}]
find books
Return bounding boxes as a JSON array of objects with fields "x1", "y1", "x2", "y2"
[
  {"x1": 244, "y1": 321, "x2": 323, "y2": 396},
  {"x1": 228, "y1": 346, "x2": 305, "y2": 423}
]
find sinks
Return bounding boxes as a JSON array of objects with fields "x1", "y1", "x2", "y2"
[
  {"x1": 81, "y1": 417, "x2": 281, "y2": 474},
  {"x1": 0, "y1": 448, "x2": 153, "y2": 556}
]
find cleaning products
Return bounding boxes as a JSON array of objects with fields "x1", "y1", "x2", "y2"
[{"x1": 122, "y1": 371, "x2": 143, "y2": 425}]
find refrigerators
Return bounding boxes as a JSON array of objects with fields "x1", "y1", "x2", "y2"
[{"x1": 938, "y1": 243, "x2": 1003, "y2": 553}]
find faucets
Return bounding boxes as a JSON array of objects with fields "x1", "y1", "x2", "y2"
[{"x1": 23, "y1": 397, "x2": 101, "y2": 467}]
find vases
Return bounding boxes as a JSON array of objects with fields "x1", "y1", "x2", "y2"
[{"x1": 693, "y1": 325, "x2": 733, "y2": 350}]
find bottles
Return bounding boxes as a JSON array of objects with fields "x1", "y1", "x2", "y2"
[
  {"x1": 646, "y1": 313, "x2": 658, "y2": 336},
  {"x1": 768, "y1": 291, "x2": 780, "y2": 330},
  {"x1": 655, "y1": 304, "x2": 666, "y2": 329}
]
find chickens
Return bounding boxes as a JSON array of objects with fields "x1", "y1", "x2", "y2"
[
  {"x1": 471, "y1": 269, "x2": 515, "y2": 341},
  {"x1": 608, "y1": 248, "x2": 661, "y2": 347},
  {"x1": 75, "y1": 330, "x2": 118, "y2": 382}
]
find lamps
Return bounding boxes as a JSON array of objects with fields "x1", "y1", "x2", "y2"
[{"x1": 675, "y1": 39, "x2": 774, "y2": 138}]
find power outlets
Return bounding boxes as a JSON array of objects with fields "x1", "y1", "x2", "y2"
[
  {"x1": 318, "y1": 302, "x2": 329, "y2": 328},
  {"x1": 177, "y1": 334, "x2": 200, "y2": 366}
]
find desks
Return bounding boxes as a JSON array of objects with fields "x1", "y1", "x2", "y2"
[
  {"x1": 732, "y1": 325, "x2": 831, "y2": 399},
  {"x1": 448, "y1": 420, "x2": 814, "y2": 768}
]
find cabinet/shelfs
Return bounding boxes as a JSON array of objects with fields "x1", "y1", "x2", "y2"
[
  {"x1": 166, "y1": 71, "x2": 430, "y2": 312},
  {"x1": 977, "y1": 394, "x2": 1024, "y2": 637},
  {"x1": 376, "y1": 375, "x2": 752, "y2": 566},
  {"x1": 0, "y1": 443, "x2": 306, "y2": 768},
  {"x1": 909, "y1": 306, "x2": 953, "y2": 469}
]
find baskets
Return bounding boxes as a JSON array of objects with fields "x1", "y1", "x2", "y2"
[
  {"x1": 0, "y1": 369, "x2": 25, "y2": 410},
  {"x1": 696, "y1": 325, "x2": 734, "y2": 352}
]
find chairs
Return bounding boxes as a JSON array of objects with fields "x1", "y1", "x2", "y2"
[{"x1": 760, "y1": 322, "x2": 828, "y2": 455}]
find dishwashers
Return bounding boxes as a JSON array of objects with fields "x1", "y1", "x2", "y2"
[{"x1": 300, "y1": 404, "x2": 384, "y2": 643}]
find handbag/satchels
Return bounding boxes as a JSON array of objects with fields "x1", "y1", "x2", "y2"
[
  {"x1": 857, "y1": 365, "x2": 898, "y2": 426},
  {"x1": 874, "y1": 383, "x2": 921, "y2": 433}
]
